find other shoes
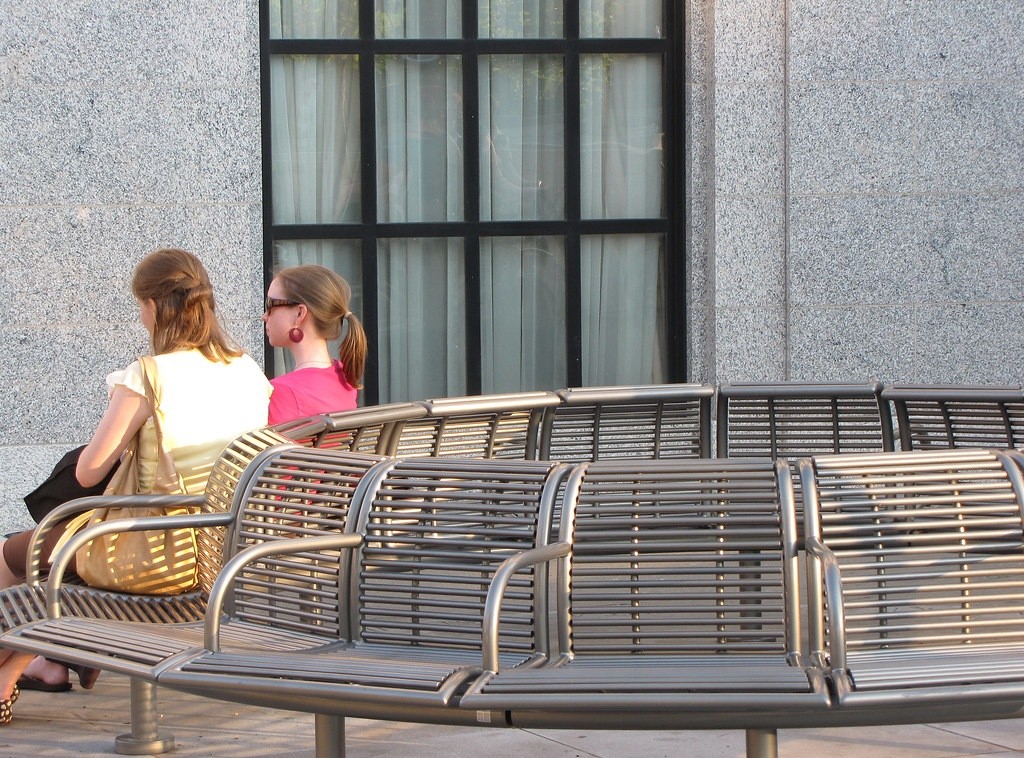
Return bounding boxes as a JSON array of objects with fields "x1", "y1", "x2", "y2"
[
  {"x1": 10, "y1": 684, "x2": 20, "y2": 703},
  {"x1": 0, "y1": 699, "x2": 12, "y2": 725}
]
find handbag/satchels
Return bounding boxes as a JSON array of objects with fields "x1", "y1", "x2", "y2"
[{"x1": 76, "y1": 357, "x2": 198, "y2": 595}]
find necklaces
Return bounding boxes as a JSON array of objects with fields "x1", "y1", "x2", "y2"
[{"x1": 295, "y1": 360, "x2": 332, "y2": 369}]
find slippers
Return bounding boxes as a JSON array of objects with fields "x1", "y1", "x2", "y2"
[{"x1": 15, "y1": 672, "x2": 73, "y2": 692}]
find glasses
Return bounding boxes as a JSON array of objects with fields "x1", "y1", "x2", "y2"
[{"x1": 265, "y1": 298, "x2": 299, "y2": 316}]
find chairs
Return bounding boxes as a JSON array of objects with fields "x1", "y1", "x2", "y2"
[{"x1": 0, "y1": 379, "x2": 1024, "y2": 758}]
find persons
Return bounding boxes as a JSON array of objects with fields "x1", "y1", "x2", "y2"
[
  {"x1": 0, "y1": 249, "x2": 274, "y2": 726},
  {"x1": 16, "y1": 265, "x2": 367, "y2": 692}
]
